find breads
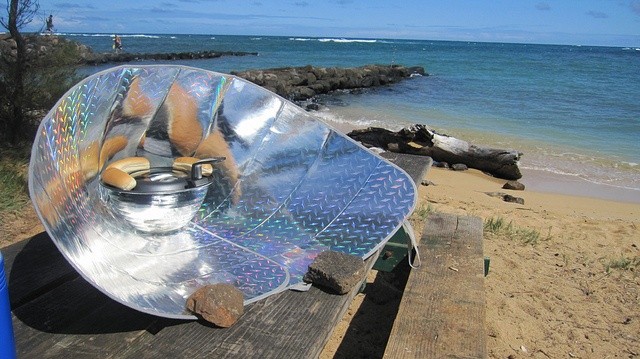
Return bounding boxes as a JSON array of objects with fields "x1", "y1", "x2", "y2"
[
  {"x1": 101, "y1": 167, "x2": 136, "y2": 191},
  {"x1": 164, "y1": 83, "x2": 202, "y2": 158},
  {"x1": 121, "y1": 81, "x2": 151, "y2": 120},
  {"x1": 98, "y1": 135, "x2": 126, "y2": 165},
  {"x1": 105, "y1": 155, "x2": 151, "y2": 177},
  {"x1": 78, "y1": 140, "x2": 99, "y2": 174},
  {"x1": 190, "y1": 126, "x2": 242, "y2": 206},
  {"x1": 173, "y1": 155, "x2": 213, "y2": 178}
]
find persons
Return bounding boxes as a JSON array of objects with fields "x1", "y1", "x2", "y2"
[
  {"x1": 45, "y1": 14, "x2": 54, "y2": 32},
  {"x1": 113, "y1": 34, "x2": 123, "y2": 54}
]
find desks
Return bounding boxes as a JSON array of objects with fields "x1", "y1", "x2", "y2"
[{"x1": 1, "y1": 148, "x2": 433, "y2": 358}]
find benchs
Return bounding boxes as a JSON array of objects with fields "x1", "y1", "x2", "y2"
[{"x1": 382, "y1": 209, "x2": 489, "y2": 359}]
